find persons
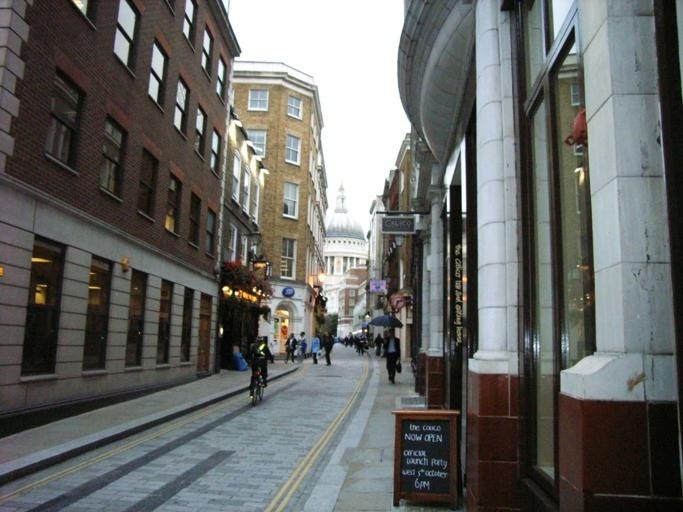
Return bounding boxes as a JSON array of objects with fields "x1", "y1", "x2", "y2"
[
  {"x1": 381, "y1": 327, "x2": 400, "y2": 384},
  {"x1": 282, "y1": 330, "x2": 386, "y2": 366},
  {"x1": 246, "y1": 336, "x2": 274, "y2": 397}
]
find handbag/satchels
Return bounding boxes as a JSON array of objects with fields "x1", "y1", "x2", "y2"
[{"x1": 396, "y1": 363, "x2": 401, "y2": 372}]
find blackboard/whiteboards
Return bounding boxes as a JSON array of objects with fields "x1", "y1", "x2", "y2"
[{"x1": 392, "y1": 410, "x2": 460, "y2": 502}]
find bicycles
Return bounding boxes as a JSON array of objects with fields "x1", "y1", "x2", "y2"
[{"x1": 249, "y1": 356, "x2": 273, "y2": 407}]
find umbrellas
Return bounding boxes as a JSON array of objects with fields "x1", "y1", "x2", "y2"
[{"x1": 367, "y1": 314, "x2": 403, "y2": 329}]
